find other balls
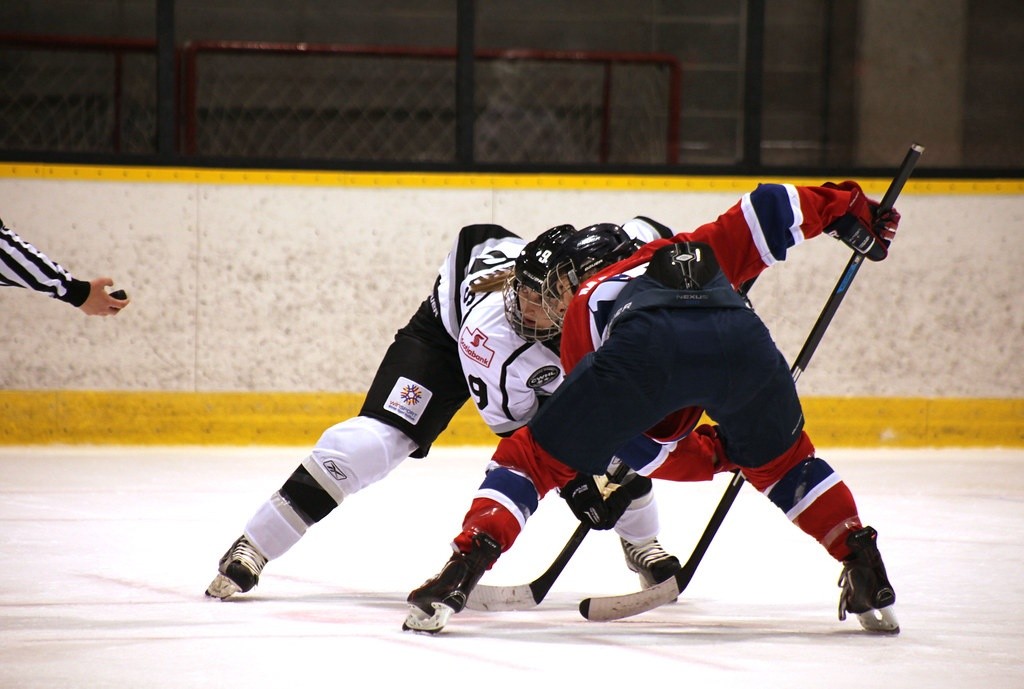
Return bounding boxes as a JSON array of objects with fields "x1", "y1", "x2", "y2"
[{"x1": 108, "y1": 288, "x2": 127, "y2": 302}]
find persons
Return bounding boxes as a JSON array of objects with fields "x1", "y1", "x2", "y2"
[
  {"x1": 405, "y1": 180, "x2": 902, "y2": 634},
  {"x1": 206, "y1": 217, "x2": 681, "y2": 603},
  {"x1": 0, "y1": 218, "x2": 130, "y2": 317}
]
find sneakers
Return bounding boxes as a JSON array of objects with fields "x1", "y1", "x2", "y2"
[
  {"x1": 621, "y1": 535, "x2": 681, "y2": 601},
  {"x1": 204, "y1": 534, "x2": 268, "y2": 603},
  {"x1": 838, "y1": 526, "x2": 899, "y2": 633},
  {"x1": 402, "y1": 533, "x2": 501, "y2": 636}
]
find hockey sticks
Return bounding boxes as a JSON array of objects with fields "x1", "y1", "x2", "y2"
[
  {"x1": 578, "y1": 138, "x2": 928, "y2": 623},
  {"x1": 466, "y1": 461, "x2": 630, "y2": 614}
]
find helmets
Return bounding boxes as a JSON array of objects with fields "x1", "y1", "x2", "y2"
[
  {"x1": 542, "y1": 224, "x2": 638, "y2": 332},
  {"x1": 502, "y1": 224, "x2": 577, "y2": 343}
]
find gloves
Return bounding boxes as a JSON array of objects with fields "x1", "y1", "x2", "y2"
[
  {"x1": 559, "y1": 477, "x2": 631, "y2": 531},
  {"x1": 820, "y1": 180, "x2": 900, "y2": 261},
  {"x1": 694, "y1": 424, "x2": 740, "y2": 473}
]
